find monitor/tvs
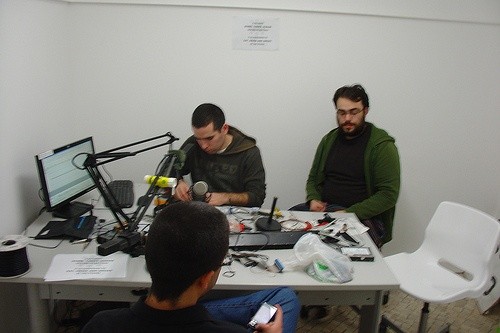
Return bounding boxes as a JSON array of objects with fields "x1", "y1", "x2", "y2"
[{"x1": 37, "y1": 135, "x2": 100, "y2": 219}]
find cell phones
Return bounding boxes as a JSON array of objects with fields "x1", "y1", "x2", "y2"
[{"x1": 248, "y1": 302, "x2": 277, "y2": 330}]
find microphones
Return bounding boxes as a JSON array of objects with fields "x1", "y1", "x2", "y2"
[{"x1": 192, "y1": 181, "x2": 208, "y2": 202}]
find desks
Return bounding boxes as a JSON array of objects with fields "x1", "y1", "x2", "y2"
[{"x1": 0, "y1": 182, "x2": 399, "y2": 333}]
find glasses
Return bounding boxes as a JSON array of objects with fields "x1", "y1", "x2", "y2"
[{"x1": 337, "y1": 107, "x2": 366, "y2": 116}]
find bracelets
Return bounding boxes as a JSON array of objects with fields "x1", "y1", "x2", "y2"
[{"x1": 228, "y1": 193, "x2": 231, "y2": 205}]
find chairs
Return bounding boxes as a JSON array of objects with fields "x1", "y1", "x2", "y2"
[{"x1": 384, "y1": 200, "x2": 500, "y2": 333}]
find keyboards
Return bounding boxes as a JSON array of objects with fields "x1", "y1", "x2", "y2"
[{"x1": 105, "y1": 179, "x2": 134, "y2": 208}]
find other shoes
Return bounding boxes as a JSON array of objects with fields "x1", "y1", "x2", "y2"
[{"x1": 315, "y1": 306, "x2": 338, "y2": 321}]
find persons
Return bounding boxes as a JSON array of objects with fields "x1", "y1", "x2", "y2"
[
  {"x1": 286, "y1": 83, "x2": 401, "y2": 249},
  {"x1": 164, "y1": 103, "x2": 267, "y2": 208},
  {"x1": 83, "y1": 200, "x2": 300, "y2": 333}
]
